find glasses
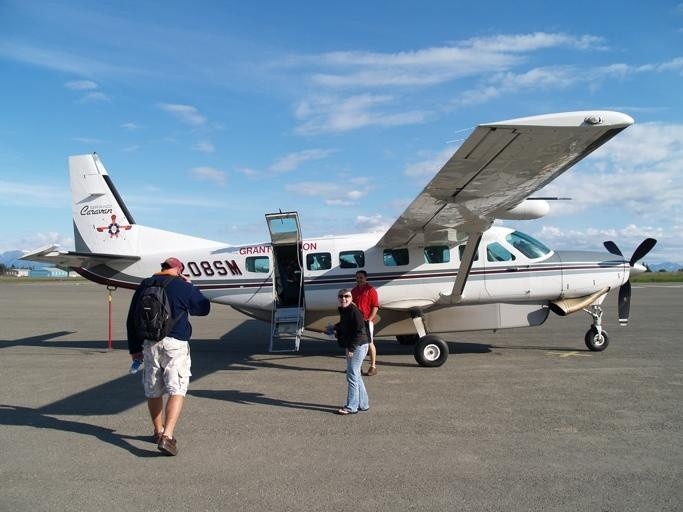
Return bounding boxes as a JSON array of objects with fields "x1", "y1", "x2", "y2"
[
  {"x1": 338, "y1": 295, "x2": 351, "y2": 298},
  {"x1": 357, "y1": 277, "x2": 363, "y2": 279}
]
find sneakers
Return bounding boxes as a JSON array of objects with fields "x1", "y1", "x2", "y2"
[
  {"x1": 367, "y1": 367, "x2": 377, "y2": 376},
  {"x1": 153, "y1": 431, "x2": 178, "y2": 456},
  {"x1": 338, "y1": 407, "x2": 369, "y2": 415}
]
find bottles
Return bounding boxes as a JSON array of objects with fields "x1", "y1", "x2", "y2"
[
  {"x1": 129, "y1": 357, "x2": 143, "y2": 375},
  {"x1": 326, "y1": 321, "x2": 335, "y2": 339}
]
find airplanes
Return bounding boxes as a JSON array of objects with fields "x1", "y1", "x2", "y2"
[{"x1": 18, "y1": 110, "x2": 657, "y2": 367}]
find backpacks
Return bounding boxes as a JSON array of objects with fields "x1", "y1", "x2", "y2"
[{"x1": 134, "y1": 276, "x2": 185, "y2": 342}]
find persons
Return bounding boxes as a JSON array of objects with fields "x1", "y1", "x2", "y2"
[
  {"x1": 126, "y1": 256, "x2": 201, "y2": 455},
  {"x1": 285, "y1": 261, "x2": 300, "y2": 283},
  {"x1": 351, "y1": 270, "x2": 380, "y2": 376},
  {"x1": 334, "y1": 289, "x2": 370, "y2": 415}
]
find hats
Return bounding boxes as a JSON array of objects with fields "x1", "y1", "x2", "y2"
[{"x1": 160, "y1": 258, "x2": 183, "y2": 271}]
